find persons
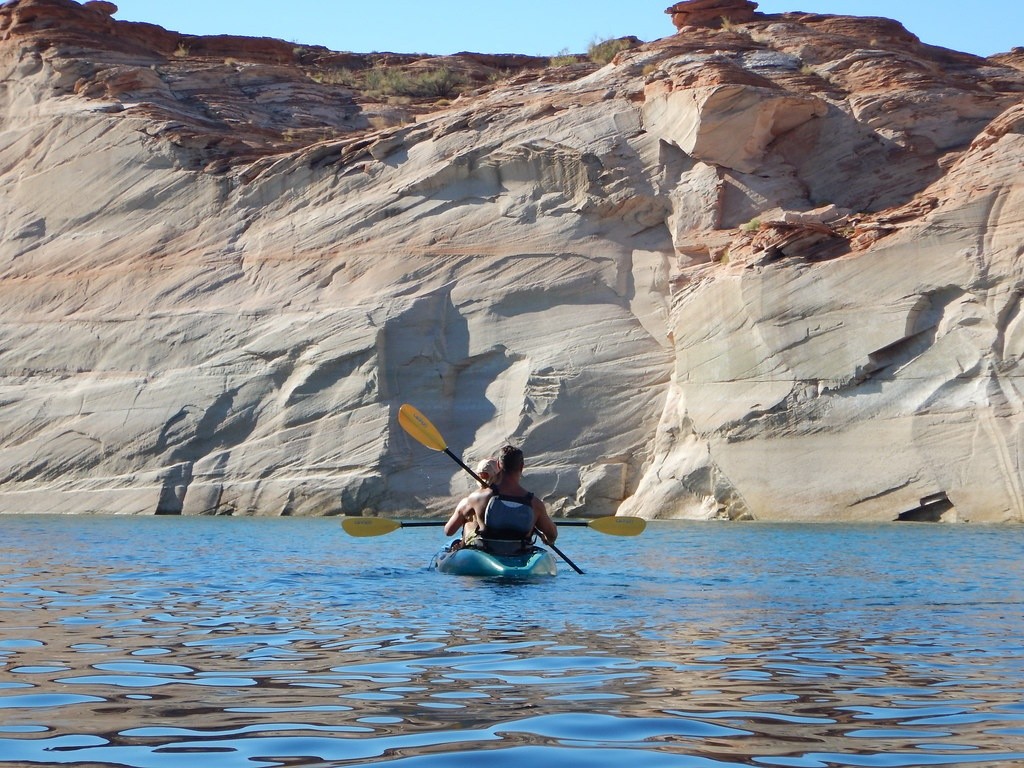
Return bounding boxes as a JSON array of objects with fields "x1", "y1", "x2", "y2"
[
  {"x1": 444, "y1": 459, "x2": 499, "y2": 544},
  {"x1": 451, "y1": 445, "x2": 558, "y2": 555}
]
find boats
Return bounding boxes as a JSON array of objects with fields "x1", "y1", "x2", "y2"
[{"x1": 429, "y1": 533, "x2": 555, "y2": 576}]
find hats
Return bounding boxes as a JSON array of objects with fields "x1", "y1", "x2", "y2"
[{"x1": 476, "y1": 458, "x2": 499, "y2": 475}]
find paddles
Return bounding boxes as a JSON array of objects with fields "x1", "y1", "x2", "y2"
[
  {"x1": 340, "y1": 515, "x2": 649, "y2": 539},
  {"x1": 395, "y1": 400, "x2": 587, "y2": 577}
]
